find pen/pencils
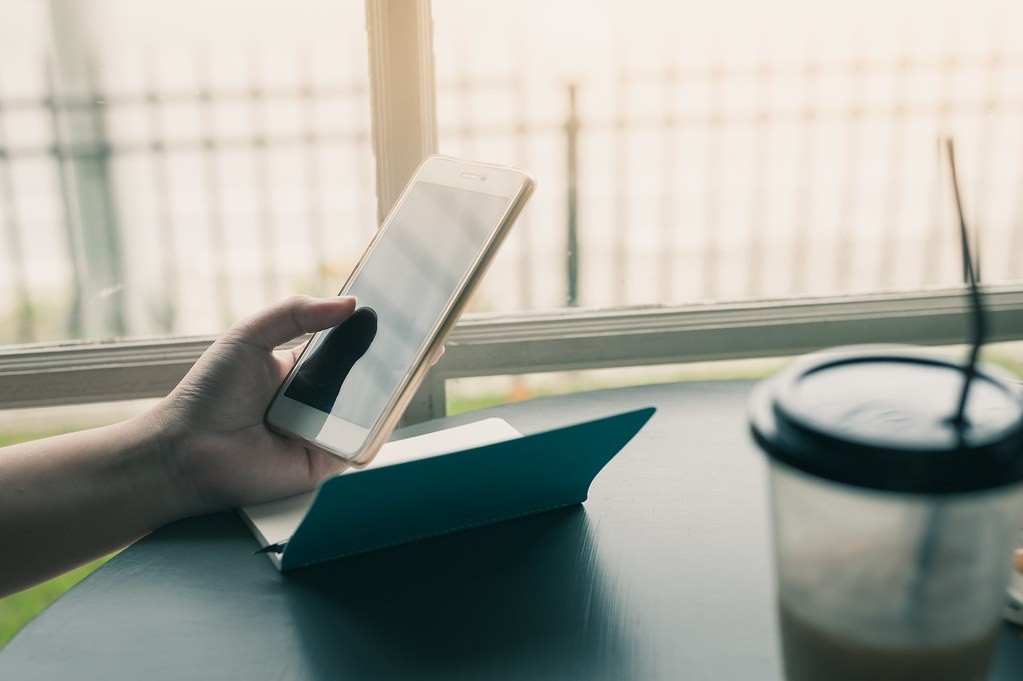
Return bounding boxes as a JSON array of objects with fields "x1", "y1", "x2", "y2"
[{"x1": 251, "y1": 539, "x2": 289, "y2": 556}]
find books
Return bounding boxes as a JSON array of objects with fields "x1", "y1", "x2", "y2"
[{"x1": 239, "y1": 404, "x2": 657, "y2": 574}]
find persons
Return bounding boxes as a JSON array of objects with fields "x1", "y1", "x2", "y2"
[{"x1": 0, "y1": 292, "x2": 445, "y2": 600}]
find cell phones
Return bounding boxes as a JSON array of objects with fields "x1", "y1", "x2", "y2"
[{"x1": 264, "y1": 156, "x2": 536, "y2": 468}]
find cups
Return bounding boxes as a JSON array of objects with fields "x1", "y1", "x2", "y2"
[{"x1": 748, "y1": 343, "x2": 1023, "y2": 681}]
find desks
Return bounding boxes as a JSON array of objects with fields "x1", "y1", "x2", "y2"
[{"x1": 0, "y1": 377, "x2": 1023, "y2": 681}]
